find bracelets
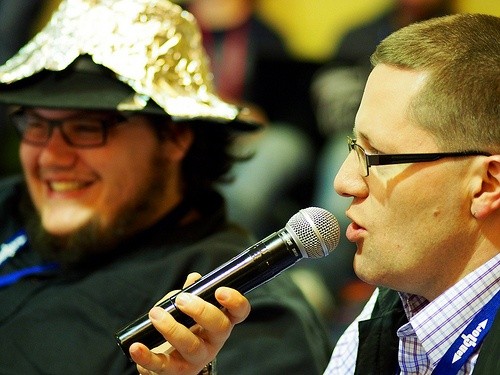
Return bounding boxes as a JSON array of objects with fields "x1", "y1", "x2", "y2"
[{"x1": 202, "y1": 361, "x2": 213, "y2": 375}]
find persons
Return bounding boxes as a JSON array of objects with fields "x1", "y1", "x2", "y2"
[
  {"x1": 322, "y1": 14, "x2": 500, "y2": 375},
  {"x1": 0, "y1": 0, "x2": 461, "y2": 375}
]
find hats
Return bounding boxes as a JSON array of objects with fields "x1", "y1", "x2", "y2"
[{"x1": 0, "y1": 0, "x2": 264, "y2": 133}]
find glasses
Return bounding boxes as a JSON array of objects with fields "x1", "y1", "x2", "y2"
[
  {"x1": 347, "y1": 136, "x2": 493, "y2": 177},
  {"x1": 9, "y1": 105, "x2": 136, "y2": 148}
]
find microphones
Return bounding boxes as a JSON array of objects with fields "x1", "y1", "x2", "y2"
[{"x1": 114, "y1": 207, "x2": 341, "y2": 363}]
